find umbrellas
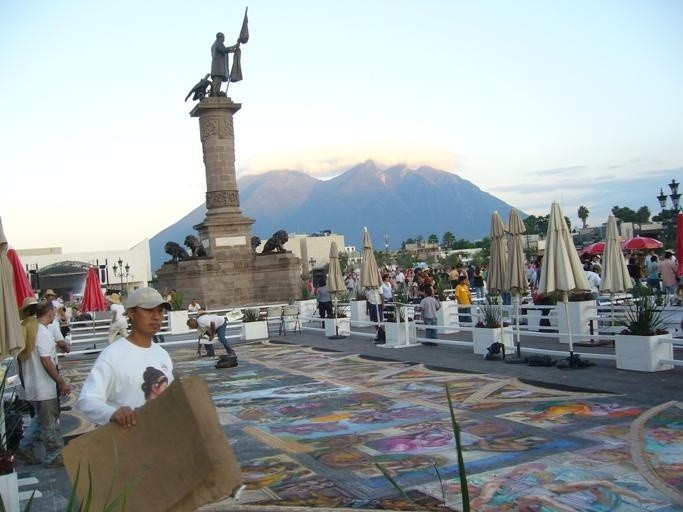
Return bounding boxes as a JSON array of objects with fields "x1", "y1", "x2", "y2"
[
  {"x1": 672, "y1": 209, "x2": 682, "y2": 286},
  {"x1": 324, "y1": 238, "x2": 347, "y2": 341},
  {"x1": 4, "y1": 242, "x2": 35, "y2": 312},
  {"x1": 484, "y1": 209, "x2": 513, "y2": 362},
  {"x1": 357, "y1": 225, "x2": 387, "y2": 345},
  {"x1": 620, "y1": 234, "x2": 664, "y2": 253},
  {"x1": 581, "y1": 242, "x2": 606, "y2": 258},
  {"x1": 0, "y1": 218, "x2": 23, "y2": 366},
  {"x1": 79, "y1": 262, "x2": 109, "y2": 348},
  {"x1": 595, "y1": 207, "x2": 634, "y2": 327},
  {"x1": 533, "y1": 200, "x2": 596, "y2": 369},
  {"x1": 500, "y1": 207, "x2": 535, "y2": 363}
]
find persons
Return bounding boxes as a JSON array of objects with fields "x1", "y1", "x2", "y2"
[
  {"x1": 15, "y1": 284, "x2": 92, "y2": 469},
  {"x1": 103, "y1": 292, "x2": 129, "y2": 345},
  {"x1": 208, "y1": 32, "x2": 239, "y2": 96},
  {"x1": 72, "y1": 285, "x2": 175, "y2": 431},
  {"x1": 185, "y1": 314, "x2": 237, "y2": 360},
  {"x1": 188, "y1": 297, "x2": 203, "y2": 313},
  {"x1": 141, "y1": 366, "x2": 168, "y2": 404}
]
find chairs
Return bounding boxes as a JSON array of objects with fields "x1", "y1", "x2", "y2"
[
  {"x1": 279, "y1": 304, "x2": 302, "y2": 334},
  {"x1": 262, "y1": 305, "x2": 286, "y2": 335}
]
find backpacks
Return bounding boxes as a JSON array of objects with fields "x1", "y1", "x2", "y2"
[{"x1": 216, "y1": 356, "x2": 238, "y2": 369}]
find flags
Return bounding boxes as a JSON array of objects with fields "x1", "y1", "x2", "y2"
[{"x1": 227, "y1": 5, "x2": 251, "y2": 85}]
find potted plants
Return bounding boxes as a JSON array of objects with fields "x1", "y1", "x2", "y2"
[
  {"x1": 385, "y1": 299, "x2": 418, "y2": 346},
  {"x1": 613, "y1": 279, "x2": 675, "y2": 372},
  {"x1": 326, "y1": 307, "x2": 351, "y2": 337},
  {"x1": 166, "y1": 288, "x2": 189, "y2": 336},
  {"x1": 472, "y1": 297, "x2": 515, "y2": 356},
  {"x1": 556, "y1": 289, "x2": 600, "y2": 345},
  {"x1": 0, "y1": 354, "x2": 43, "y2": 511},
  {"x1": 347, "y1": 289, "x2": 374, "y2": 328},
  {"x1": 432, "y1": 273, "x2": 459, "y2": 334},
  {"x1": 240, "y1": 307, "x2": 268, "y2": 341}
]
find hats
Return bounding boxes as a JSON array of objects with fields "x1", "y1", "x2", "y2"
[
  {"x1": 126, "y1": 287, "x2": 172, "y2": 312},
  {"x1": 17, "y1": 296, "x2": 39, "y2": 310},
  {"x1": 665, "y1": 249, "x2": 676, "y2": 255},
  {"x1": 105, "y1": 293, "x2": 121, "y2": 304},
  {"x1": 17, "y1": 316, "x2": 39, "y2": 362},
  {"x1": 42, "y1": 289, "x2": 57, "y2": 296}
]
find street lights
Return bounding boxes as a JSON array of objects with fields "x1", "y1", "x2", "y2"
[
  {"x1": 383, "y1": 231, "x2": 389, "y2": 262},
  {"x1": 657, "y1": 177, "x2": 680, "y2": 251},
  {"x1": 112, "y1": 256, "x2": 131, "y2": 294},
  {"x1": 308, "y1": 257, "x2": 316, "y2": 270}
]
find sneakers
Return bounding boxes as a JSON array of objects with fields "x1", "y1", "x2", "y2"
[{"x1": 14, "y1": 448, "x2": 66, "y2": 468}]
